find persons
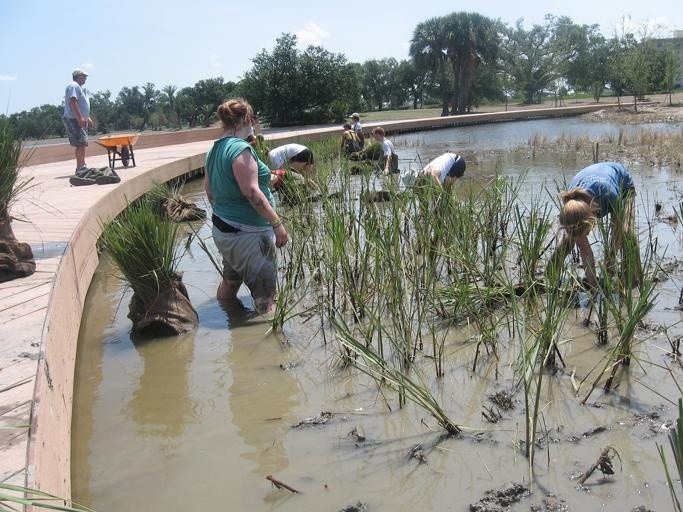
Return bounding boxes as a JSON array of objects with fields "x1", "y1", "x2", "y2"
[
  {"x1": 61, "y1": 70, "x2": 94, "y2": 176},
  {"x1": 373, "y1": 127, "x2": 400, "y2": 176},
  {"x1": 348, "y1": 112, "x2": 364, "y2": 149},
  {"x1": 245, "y1": 116, "x2": 314, "y2": 212},
  {"x1": 544, "y1": 160, "x2": 641, "y2": 293},
  {"x1": 204, "y1": 97, "x2": 289, "y2": 315},
  {"x1": 340, "y1": 122, "x2": 362, "y2": 155},
  {"x1": 401, "y1": 151, "x2": 466, "y2": 223}
]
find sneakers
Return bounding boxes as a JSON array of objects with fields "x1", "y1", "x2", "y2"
[{"x1": 74, "y1": 166, "x2": 88, "y2": 175}]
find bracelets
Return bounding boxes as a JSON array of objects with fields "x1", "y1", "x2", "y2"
[{"x1": 271, "y1": 218, "x2": 281, "y2": 225}]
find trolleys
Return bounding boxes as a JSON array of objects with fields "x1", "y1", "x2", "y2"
[{"x1": 94, "y1": 133, "x2": 139, "y2": 168}]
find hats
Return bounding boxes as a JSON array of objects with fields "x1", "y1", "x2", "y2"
[
  {"x1": 72, "y1": 70, "x2": 88, "y2": 76},
  {"x1": 349, "y1": 113, "x2": 359, "y2": 118}
]
